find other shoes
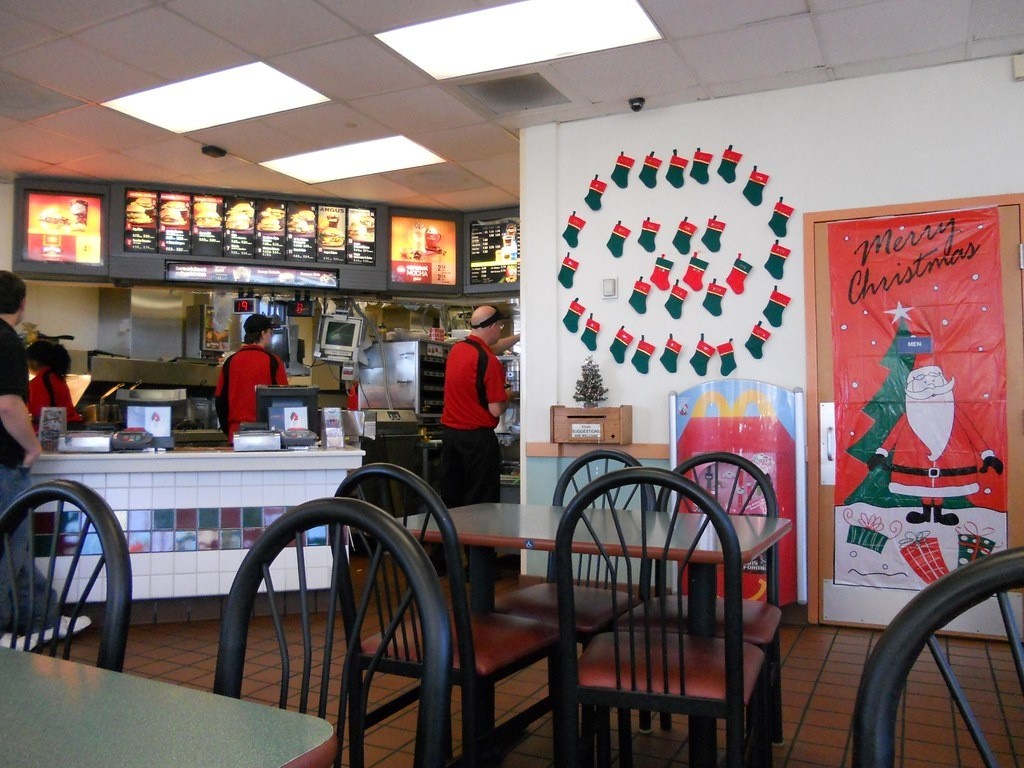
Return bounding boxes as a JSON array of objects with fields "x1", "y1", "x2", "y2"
[
  {"x1": 58, "y1": 614, "x2": 92, "y2": 638},
  {"x1": 1, "y1": 629, "x2": 55, "y2": 652}
]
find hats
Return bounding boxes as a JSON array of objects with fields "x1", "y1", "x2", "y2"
[
  {"x1": 244, "y1": 314, "x2": 281, "y2": 335},
  {"x1": 471, "y1": 307, "x2": 511, "y2": 329}
]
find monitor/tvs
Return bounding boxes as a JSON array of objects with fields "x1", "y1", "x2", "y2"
[
  {"x1": 320, "y1": 317, "x2": 361, "y2": 351},
  {"x1": 271, "y1": 329, "x2": 287, "y2": 359}
]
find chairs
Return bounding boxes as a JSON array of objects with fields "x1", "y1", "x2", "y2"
[{"x1": 0, "y1": 459, "x2": 1024, "y2": 768}]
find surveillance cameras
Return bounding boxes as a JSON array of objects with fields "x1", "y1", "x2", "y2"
[{"x1": 631, "y1": 97, "x2": 645, "y2": 112}]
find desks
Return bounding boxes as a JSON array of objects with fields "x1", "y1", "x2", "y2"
[
  {"x1": 1, "y1": 645, "x2": 343, "y2": 768},
  {"x1": 355, "y1": 500, "x2": 793, "y2": 768}
]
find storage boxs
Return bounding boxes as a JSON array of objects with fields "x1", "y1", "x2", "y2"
[{"x1": 549, "y1": 403, "x2": 632, "y2": 445}]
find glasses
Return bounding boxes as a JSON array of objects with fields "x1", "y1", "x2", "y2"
[{"x1": 492, "y1": 322, "x2": 505, "y2": 330}]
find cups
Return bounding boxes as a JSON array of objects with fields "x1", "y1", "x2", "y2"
[
  {"x1": 425, "y1": 233, "x2": 441, "y2": 249},
  {"x1": 503, "y1": 235, "x2": 512, "y2": 247},
  {"x1": 412, "y1": 228, "x2": 426, "y2": 252},
  {"x1": 68, "y1": 200, "x2": 88, "y2": 224}
]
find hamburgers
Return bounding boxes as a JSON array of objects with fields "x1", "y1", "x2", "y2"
[
  {"x1": 318, "y1": 215, "x2": 375, "y2": 247},
  {"x1": 126, "y1": 198, "x2": 316, "y2": 234}
]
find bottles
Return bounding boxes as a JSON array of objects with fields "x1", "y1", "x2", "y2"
[{"x1": 378, "y1": 322, "x2": 386, "y2": 340}]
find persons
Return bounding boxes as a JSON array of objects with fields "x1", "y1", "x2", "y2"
[
  {"x1": 26, "y1": 341, "x2": 84, "y2": 435},
  {"x1": 215, "y1": 314, "x2": 289, "y2": 447},
  {"x1": 440, "y1": 306, "x2": 520, "y2": 612},
  {"x1": 0, "y1": 270, "x2": 92, "y2": 654}
]
